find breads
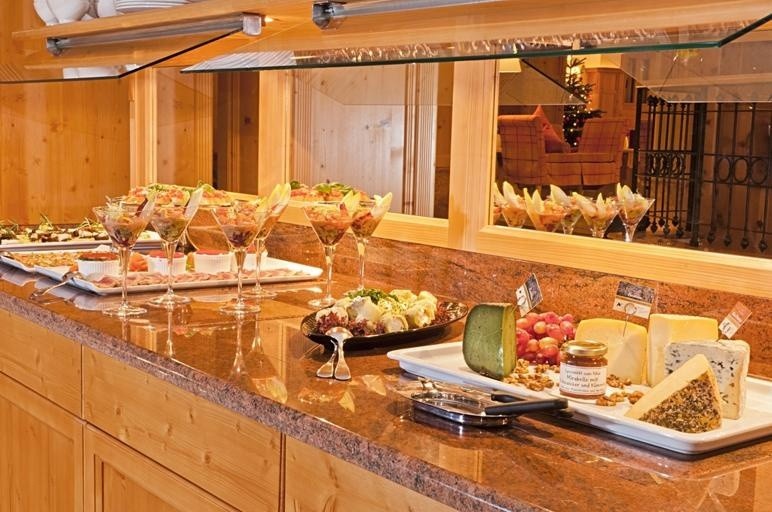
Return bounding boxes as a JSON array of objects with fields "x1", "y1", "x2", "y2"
[
  {"x1": 663, "y1": 339, "x2": 751, "y2": 420},
  {"x1": 462, "y1": 303, "x2": 516, "y2": 382},
  {"x1": 646, "y1": 313, "x2": 718, "y2": 388},
  {"x1": 574, "y1": 318, "x2": 647, "y2": 386},
  {"x1": 621, "y1": 353, "x2": 724, "y2": 435}
]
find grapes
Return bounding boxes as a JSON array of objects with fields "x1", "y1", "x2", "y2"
[{"x1": 516, "y1": 311, "x2": 576, "y2": 367}]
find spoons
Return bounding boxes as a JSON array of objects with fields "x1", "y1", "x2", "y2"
[{"x1": 323, "y1": 326, "x2": 352, "y2": 383}]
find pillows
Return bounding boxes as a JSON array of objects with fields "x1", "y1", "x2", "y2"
[{"x1": 531, "y1": 105, "x2": 568, "y2": 151}]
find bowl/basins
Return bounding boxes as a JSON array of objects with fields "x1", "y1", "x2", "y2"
[
  {"x1": 47, "y1": 2, "x2": 90, "y2": 24},
  {"x1": 33, "y1": 2, "x2": 58, "y2": 27}
]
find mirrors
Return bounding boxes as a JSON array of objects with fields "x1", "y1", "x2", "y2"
[
  {"x1": 489, "y1": 43, "x2": 772, "y2": 259},
  {"x1": 156, "y1": 67, "x2": 260, "y2": 194},
  {"x1": 288, "y1": 61, "x2": 454, "y2": 219}
]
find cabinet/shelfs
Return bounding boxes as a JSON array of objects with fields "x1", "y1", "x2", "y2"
[{"x1": 0, "y1": 306, "x2": 457, "y2": 512}]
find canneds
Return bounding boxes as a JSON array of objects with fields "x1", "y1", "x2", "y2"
[{"x1": 559, "y1": 339, "x2": 609, "y2": 400}]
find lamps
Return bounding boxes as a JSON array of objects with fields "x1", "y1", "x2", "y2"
[
  {"x1": 47, "y1": 15, "x2": 262, "y2": 55},
  {"x1": 312, "y1": 0, "x2": 490, "y2": 29}
]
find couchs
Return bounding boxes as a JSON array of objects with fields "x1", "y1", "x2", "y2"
[{"x1": 498, "y1": 114, "x2": 631, "y2": 198}]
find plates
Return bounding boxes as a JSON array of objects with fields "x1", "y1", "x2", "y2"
[
  {"x1": 301, "y1": 294, "x2": 469, "y2": 342},
  {"x1": 114, "y1": 1, "x2": 186, "y2": 11}
]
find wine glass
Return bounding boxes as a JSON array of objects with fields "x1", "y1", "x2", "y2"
[
  {"x1": 307, "y1": 197, "x2": 350, "y2": 312},
  {"x1": 92, "y1": 204, "x2": 150, "y2": 317},
  {"x1": 348, "y1": 192, "x2": 383, "y2": 299},
  {"x1": 491, "y1": 186, "x2": 656, "y2": 247},
  {"x1": 244, "y1": 204, "x2": 288, "y2": 299},
  {"x1": 213, "y1": 205, "x2": 264, "y2": 319},
  {"x1": 148, "y1": 199, "x2": 197, "y2": 308}
]
